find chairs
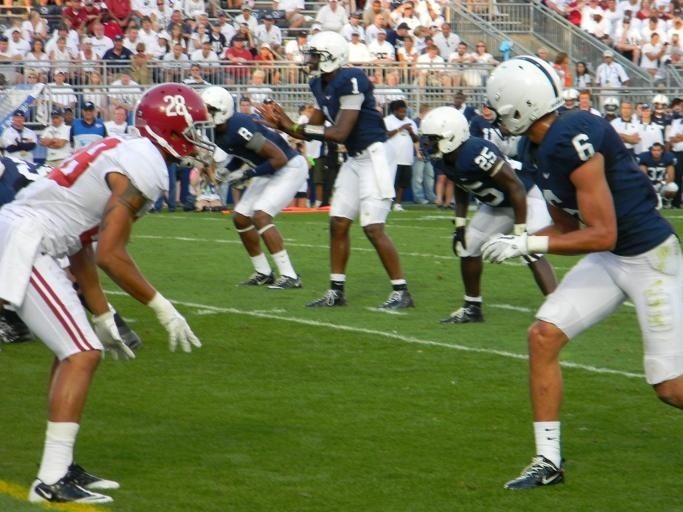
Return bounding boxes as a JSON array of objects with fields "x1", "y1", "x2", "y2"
[
  {"x1": 1, "y1": 1, "x2": 524, "y2": 86},
  {"x1": 547, "y1": 1, "x2": 683, "y2": 89}
]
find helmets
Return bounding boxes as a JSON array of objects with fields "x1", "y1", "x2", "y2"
[
  {"x1": 603, "y1": 97, "x2": 619, "y2": 115},
  {"x1": 299, "y1": 31, "x2": 351, "y2": 79},
  {"x1": 486, "y1": 54, "x2": 565, "y2": 136},
  {"x1": 135, "y1": 82, "x2": 234, "y2": 167},
  {"x1": 418, "y1": 106, "x2": 471, "y2": 163},
  {"x1": 564, "y1": 89, "x2": 580, "y2": 109},
  {"x1": 652, "y1": 95, "x2": 669, "y2": 107}
]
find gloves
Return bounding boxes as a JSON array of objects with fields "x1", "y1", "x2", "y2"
[
  {"x1": 453, "y1": 226, "x2": 466, "y2": 257},
  {"x1": 214, "y1": 168, "x2": 229, "y2": 182},
  {"x1": 479, "y1": 232, "x2": 528, "y2": 264},
  {"x1": 225, "y1": 170, "x2": 243, "y2": 183},
  {"x1": 91, "y1": 309, "x2": 136, "y2": 361},
  {"x1": 149, "y1": 290, "x2": 202, "y2": 354}
]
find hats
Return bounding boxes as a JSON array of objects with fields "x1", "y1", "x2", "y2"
[
  {"x1": 211, "y1": 5, "x2": 274, "y2": 26},
  {"x1": 14, "y1": 101, "x2": 94, "y2": 118},
  {"x1": 603, "y1": 51, "x2": 614, "y2": 58},
  {"x1": 398, "y1": 23, "x2": 411, "y2": 30}
]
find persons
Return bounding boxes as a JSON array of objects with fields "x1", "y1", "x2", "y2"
[
  {"x1": 250, "y1": 24, "x2": 416, "y2": 314},
  {"x1": 542, "y1": 1, "x2": 683, "y2": 77},
  {"x1": 0, "y1": 80, "x2": 217, "y2": 507},
  {"x1": 197, "y1": 81, "x2": 311, "y2": 292},
  {"x1": 478, "y1": 53, "x2": 683, "y2": 493},
  {"x1": 0, "y1": 153, "x2": 55, "y2": 349},
  {"x1": 1, "y1": 0, "x2": 520, "y2": 212},
  {"x1": 536, "y1": 48, "x2": 682, "y2": 209},
  {"x1": 417, "y1": 103, "x2": 562, "y2": 331}
]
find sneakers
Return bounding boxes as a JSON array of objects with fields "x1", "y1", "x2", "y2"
[
  {"x1": 267, "y1": 273, "x2": 303, "y2": 289},
  {"x1": 237, "y1": 270, "x2": 276, "y2": 285},
  {"x1": 439, "y1": 304, "x2": 484, "y2": 325},
  {"x1": 28, "y1": 473, "x2": 114, "y2": 506},
  {"x1": 393, "y1": 203, "x2": 404, "y2": 211},
  {"x1": 0, "y1": 318, "x2": 35, "y2": 344},
  {"x1": 502, "y1": 452, "x2": 566, "y2": 490},
  {"x1": 305, "y1": 290, "x2": 345, "y2": 308},
  {"x1": 69, "y1": 464, "x2": 120, "y2": 490},
  {"x1": 378, "y1": 289, "x2": 415, "y2": 311},
  {"x1": 113, "y1": 310, "x2": 141, "y2": 350}
]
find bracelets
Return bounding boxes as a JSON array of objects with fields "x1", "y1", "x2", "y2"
[
  {"x1": 290, "y1": 120, "x2": 301, "y2": 132},
  {"x1": 514, "y1": 222, "x2": 528, "y2": 236},
  {"x1": 453, "y1": 216, "x2": 466, "y2": 229}
]
